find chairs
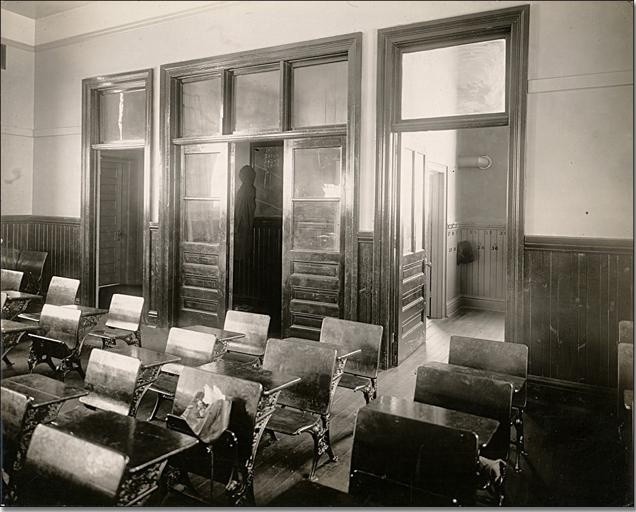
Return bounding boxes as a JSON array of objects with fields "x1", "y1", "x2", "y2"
[
  {"x1": 0, "y1": 244, "x2": 382, "y2": 507},
  {"x1": 616, "y1": 320, "x2": 634, "y2": 507},
  {"x1": 347, "y1": 331, "x2": 529, "y2": 506}
]
